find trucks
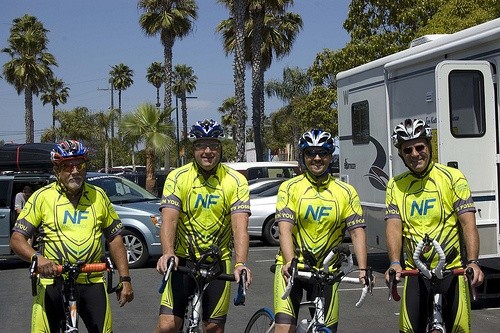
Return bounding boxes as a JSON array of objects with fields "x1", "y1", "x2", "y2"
[{"x1": 337, "y1": 18, "x2": 500, "y2": 310}]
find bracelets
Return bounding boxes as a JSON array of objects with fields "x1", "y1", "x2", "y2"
[
  {"x1": 390, "y1": 261, "x2": 400, "y2": 265},
  {"x1": 234, "y1": 262, "x2": 247, "y2": 269},
  {"x1": 31, "y1": 251, "x2": 42, "y2": 263},
  {"x1": 287, "y1": 261, "x2": 291, "y2": 264},
  {"x1": 466, "y1": 260, "x2": 480, "y2": 266},
  {"x1": 119, "y1": 276, "x2": 131, "y2": 283}
]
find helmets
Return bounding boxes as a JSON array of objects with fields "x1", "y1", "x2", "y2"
[
  {"x1": 298, "y1": 129, "x2": 335, "y2": 153},
  {"x1": 50, "y1": 140, "x2": 89, "y2": 166},
  {"x1": 189, "y1": 119, "x2": 226, "y2": 142},
  {"x1": 393, "y1": 118, "x2": 432, "y2": 149}
]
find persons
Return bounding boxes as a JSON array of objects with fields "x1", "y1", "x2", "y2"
[
  {"x1": 273, "y1": 128, "x2": 375, "y2": 333},
  {"x1": 15, "y1": 185, "x2": 34, "y2": 248},
  {"x1": 152, "y1": 119, "x2": 253, "y2": 333},
  {"x1": 9, "y1": 140, "x2": 134, "y2": 333},
  {"x1": 384, "y1": 118, "x2": 482, "y2": 333}
]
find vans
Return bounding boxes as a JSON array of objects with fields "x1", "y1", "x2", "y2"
[
  {"x1": 0, "y1": 172, "x2": 163, "y2": 269},
  {"x1": 223, "y1": 162, "x2": 302, "y2": 181}
]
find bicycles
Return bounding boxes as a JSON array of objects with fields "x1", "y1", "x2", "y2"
[
  {"x1": 388, "y1": 238, "x2": 476, "y2": 333},
  {"x1": 29, "y1": 254, "x2": 123, "y2": 333},
  {"x1": 244, "y1": 246, "x2": 373, "y2": 333},
  {"x1": 158, "y1": 250, "x2": 247, "y2": 333}
]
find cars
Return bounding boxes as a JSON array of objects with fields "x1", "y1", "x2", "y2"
[
  {"x1": 248, "y1": 178, "x2": 293, "y2": 247},
  {"x1": 121, "y1": 172, "x2": 167, "y2": 198}
]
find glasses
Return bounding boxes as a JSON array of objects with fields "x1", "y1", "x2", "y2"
[
  {"x1": 303, "y1": 149, "x2": 330, "y2": 158},
  {"x1": 192, "y1": 140, "x2": 222, "y2": 151},
  {"x1": 402, "y1": 144, "x2": 428, "y2": 155},
  {"x1": 58, "y1": 160, "x2": 87, "y2": 172},
  {"x1": 25, "y1": 193, "x2": 30, "y2": 197}
]
